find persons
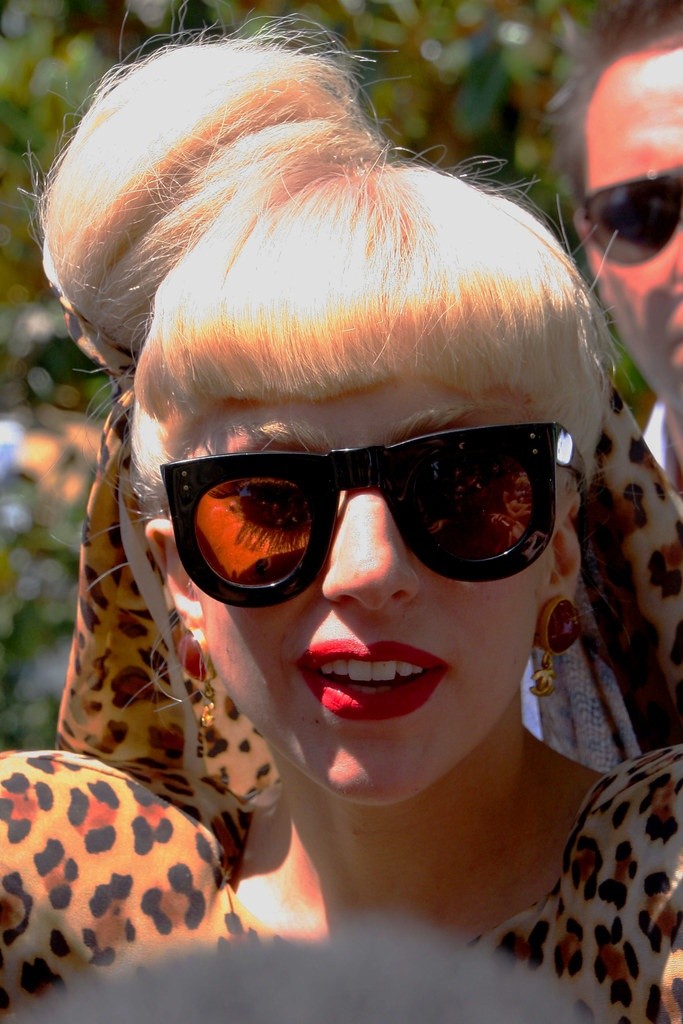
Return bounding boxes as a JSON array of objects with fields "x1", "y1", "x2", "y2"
[
  {"x1": 0, "y1": 5, "x2": 681, "y2": 1024},
  {"x1": 519, "y1": 4, "x2": 683, "y2": 769}
]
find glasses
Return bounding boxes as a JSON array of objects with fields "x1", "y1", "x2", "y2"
[
  {"x1": 158, "y1": 421, "x2": 584, "y2": 609},
  {"x1": 580, "y1": 159, "x2": 681, "y2": 265}
]
